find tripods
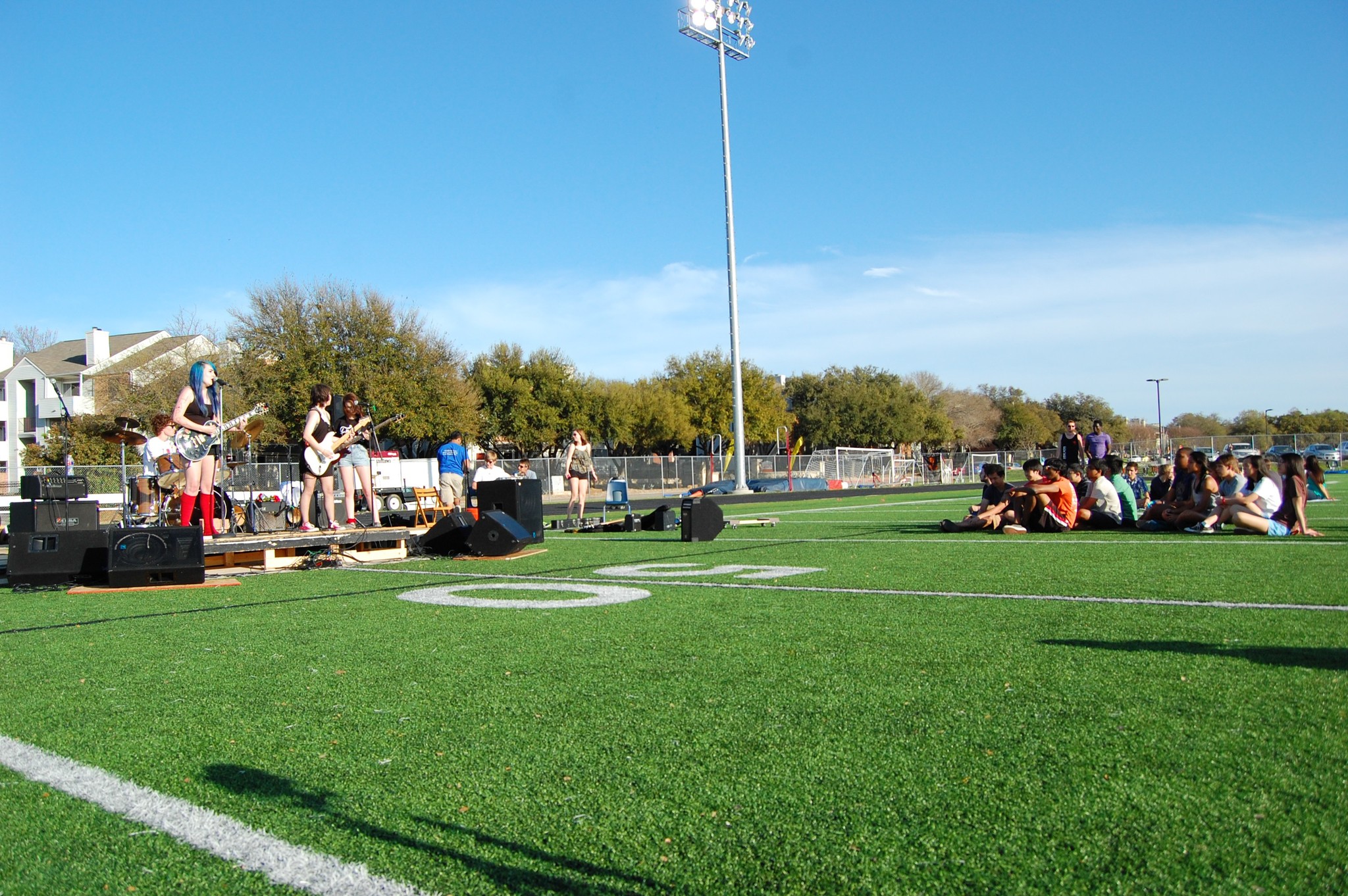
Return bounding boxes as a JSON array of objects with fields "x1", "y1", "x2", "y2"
[
  {"x1": 227, "y1": 432, "x2": 273, "y2": 536},
  {"x1": 105, "y1": 441, "x2": 137, "y2": 528}
]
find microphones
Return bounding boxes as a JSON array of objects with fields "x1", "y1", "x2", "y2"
[
  {"x1": 353, "y1": 400, "x2": 374, "y2": 407},
  {"x1": 50, "y1": 377, "x2": 60, "y2": 396}
]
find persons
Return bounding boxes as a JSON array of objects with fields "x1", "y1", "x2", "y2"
[
  {"x1": 939, "y1": 465, "x2": 1017, "y2": 532},
  {"x1": 1136, "y1": 447, "x2": 1196, "y2": 529},
  {"x1": 1161, "y1": 451, "x2": 1220, "y2": 532},
  {"x1": 1072, "y1": 458, "x2": 1122, "y2": 529},
  {"x1": 173, "y1": 360, "x2": 248, "y2": 542},
  {"x1": 143, "y1": 412, "x2": 178, "y2": 476},
  {"x1": 564, "y1": 429, "x2": 598, "y2": 519},
  {"x1": 1184, "y1": 455, "x2": 1281, "y2": 535},
  {"x1": 34, "y1": 450, "x2": 75, "y2": 478},
  {"x1": 512, "y1": 459, "x2": 537, "y2": 480},
  {"x1": 1103, "y1": 455, "x2": 1138, "y2": 528},
  {"x1": 1229, "y1": 453, "x2": 1325, "y2": 538},
  {"x1": 335, "y1": 392, "x2": 384, "y2": 530},
  {"x1": 437, "y1": 431, "x2": 470, "y2": 517},
  {"x1": 962, "y1": 417, "x2": 1336, "y2": 524},
  {"x1": 298, "y1": 383, "x2": 346, "y2": 532},
  {"x1": 472, "y1": 450, "x2": 512, "y2": 491},
  {"x1": 1002, "y1": 458, "x2": 1078, "y2": 534}
]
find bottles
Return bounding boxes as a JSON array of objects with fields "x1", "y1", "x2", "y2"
[{"x1": 67, "y1": 455, "x2": 74, "y2": 475}]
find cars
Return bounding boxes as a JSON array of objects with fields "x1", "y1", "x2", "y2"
[
  {"x1": 946, "y1": 457, "x2": 1055, "y2": 477},
  {"x1": 1112, "y1": 451, "x2": 1173, "y2": 464}
]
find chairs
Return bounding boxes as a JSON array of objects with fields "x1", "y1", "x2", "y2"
[
  {"x1": 412, "y1": 485, "x2": 453, "y2": 528},
  {"x1": 1332, "y1": 460, "x2": 1340, "y2": 472},
  {"x1": 1324, "y1": 462, "x2": 1334, "y2": 473},
  {"x1": 603, "y1": 481, "x2": 631, "y2": 523}
]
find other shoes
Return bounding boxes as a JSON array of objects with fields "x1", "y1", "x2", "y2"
[
  {"x1": 939, "y1": 519, "x2": 960, "y2": 533},
  {"x1": 1136, "y1": 520, "x2": 1148, "y2": 528}
]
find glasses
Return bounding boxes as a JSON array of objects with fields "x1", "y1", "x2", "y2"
[
  {"x1": 1068, "y1": 425, "x2": 1075, "y2": 427},
  {"x1": 487, "y1": 461, "x2": 492, "y2": 464},
  {"x1": 1042, "y1": 465, "x2": 1059, "y2": 471},
  {"x1": 1086, "y1": 466, "x2": 1098, "y2": 471},
  {"x1": 574, "y1": 433, "x2": 577, "y2": 436},
  {"x1": 166, "y1": 424, "x2": 175, "y2": 429},
  {"x1": 518, "y1": 464, "x2": 524, "y2": 468}
]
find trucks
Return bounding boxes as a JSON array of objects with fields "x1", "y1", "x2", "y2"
[{"x1": 349, "y1": 450, "x2": 441, "y2": 510}]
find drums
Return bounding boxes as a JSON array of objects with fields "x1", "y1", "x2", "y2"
[
  {"x1": 153, "y1": 453, "x2": 186, "y2": 489},
  {"x1": 162, "y1": 485, "x2": 233, "y2": 534},
  {"x1": 127, "y1": 476, "x2": 161, "y2": 517},
  {"x1": 214, "y1": 455, "x2": 231, "y2": 487}
]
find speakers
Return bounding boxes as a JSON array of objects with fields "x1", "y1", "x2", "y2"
[
  {"x1": 107, "y1": 524, "x2": 208, "y2": 587},
  {"x1": 679, "y1": 497, "x2": 723, "y2": 543},
  {"x1": 473, "y1": 477, "x2": 546, "y2": 544}
]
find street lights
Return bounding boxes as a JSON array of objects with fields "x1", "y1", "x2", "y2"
[
  {"x1": 1265, "y1": 408, "x2": 1273, "y2": 450},
  {"x1": 677, "y1": 0, "x2": 760, "y2": 497},
  {"x1": 1147, "y1": 377, "x2": 1169, "y2": 458}
]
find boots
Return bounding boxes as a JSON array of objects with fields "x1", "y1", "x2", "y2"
[
  {"x1": 181, "y1": 491, "x2": 197, "y2": 526},
  {"x1": 200, "y1": 493, "x2": 220, "y2": 540}
]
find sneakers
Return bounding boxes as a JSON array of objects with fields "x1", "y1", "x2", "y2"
[
  {"x1": 455, "y1": 505, "x2": 461, "y2": 513},
  {"x1": 1184, "y1": 522, "x2": 1214, "y2": 534},
  {"x1": 1002, "y1": 524, "x2": 1027, "y2": 534},
  {"x1": 300, "y1": 522, "x2": 319, "y2": 533},
  {"x1": 328, "y1": 520, "x2": 346, "y2": 531},
  {"x1": 1234, "y1": 526, "x2": 1257, "y2": 535},
  {"x1": 1211, "y1": 523, "x2": 1222, "y2": 532},
  {"x1": 344, "y1": 523, "x2": 356, "y2": 530}
]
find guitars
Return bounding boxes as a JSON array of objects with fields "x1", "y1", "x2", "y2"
[
  {"x1": 331, "y1": 412, "x2": 407, "y2": 466},
  {"x1": 174, "y1": 402, "x2": 270, "y2": 467},
  {"x1": 304, "y1": 415, "x2": 371, "y2": 476}
]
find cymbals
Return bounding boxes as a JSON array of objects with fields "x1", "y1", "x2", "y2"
[
  {"x1": 224, "y1": 461, "x2": 249, "y2": 468},
  {"x1": 114, "y1": 417, "x2": 141, "y2": 428},
  {"x1": 230, "y1": 419, "x2": 265, "y2": 450},
  {"x1": 100, "y1": 430, "x2": 148, "y2": 445}
]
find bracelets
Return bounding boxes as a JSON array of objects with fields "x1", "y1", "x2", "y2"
[
  {"x1": 1222, "y1": 497, "x2": 1226, "y2": 504},
  {"x1": 1011, "y1": 487, "x2": 1014, "y2": 491}
]
